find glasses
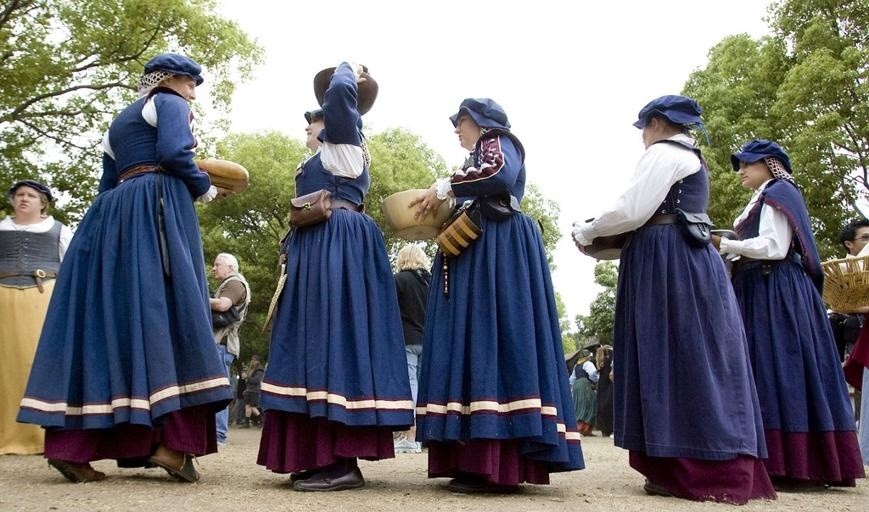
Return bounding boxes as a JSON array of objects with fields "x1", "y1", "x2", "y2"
[{"x1": 854, "y1": 233, "x2": 868, "y2": 242}]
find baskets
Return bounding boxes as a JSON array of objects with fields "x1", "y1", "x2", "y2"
[{"x1": 819, "y1": 256, "x2": 869, "y2": 313}]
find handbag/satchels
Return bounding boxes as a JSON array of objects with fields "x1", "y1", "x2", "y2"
[
  {"x1": 435, "y1": 211, "x2": 482, "y2": 259},
  {"x1": 289, "y1": 189, "x2": 332, "y2": 228},
  {"x1": 675, "y1": 208, "x2": 717, "y2": 247},
  {"x1": 212, "y1": 307, "x2": 241, "y2": 329}
]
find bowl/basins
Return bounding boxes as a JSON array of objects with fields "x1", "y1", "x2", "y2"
[
  {"x1": 707, "y1": 229, "x2": 744, "y2": 258},
  {"x1": 313, "y1": 63, "x2": 378, "y2": 116},
  {"x1": 573, "y1": 215, "x2": 626, "y2": 261},
  {"x1": 381, "y1": 187, "x2": 458, "y2": 240}
]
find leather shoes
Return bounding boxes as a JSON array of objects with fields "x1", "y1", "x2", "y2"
[
  {"x1": 289, "y1": 469, "x2": 316, "y2": 484},
  {"x1": 450, "y1": 479, "x2": 503, "y2": 495},
  {"x1": 294, "y1": 466, "x2": 364, "y2": 491}
]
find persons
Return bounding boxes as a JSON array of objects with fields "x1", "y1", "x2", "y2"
[
  {"x1": 827, "y1": 219, "x2": 868, "y2": 464},
  {"x1": 569, "y1": 345, "x2": 613, "y2": 438},
  {"x1": 257, "y1": 62, "x2": 416, "y2": 491},
  {"x1": 0, "y1": 181, "x2": 74, "y2": 454},
  {"x1": 209, "y1": 253, "x2": 251, "y2": 446},
  {"x1": 230, "y1": 355, "x2": 264, "y2": 429},
  {"x1": 407, "y1": 95, "x2": 585, "y2": 493},
  {"x1": 710, "y1": 141, "x2": 867, "y2": 490},
  {"x1": 16, "y1": 53, "x2": 233, "y2": 483},
  {"x1": 395, "y1": 245, "x2": 432, "y2": 453},
  {"x1": 571, "y1": 96, "x2": 778, "y2": 505}
]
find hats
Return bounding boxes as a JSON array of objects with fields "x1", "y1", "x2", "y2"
[
  {"x1": 450, "y1": 98, "x2": 511, "y2": 130},
  {"x1": 730, "y1": 140, "x2": 793, "y2": 172},
  {"x1": 10, "y1": 179, "x2": 53, "y2": 200},
  {"x1": 304, "y1": 109, "x2": 363, "y2": 129},
  {"x1": 144, "y1": 53, "x2": 204, "y2": 86},
  {"x1": 633, "y1": 95, "x2": 702, "y2": 130}
]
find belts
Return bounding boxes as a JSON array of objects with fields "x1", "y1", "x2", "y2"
[
  {"x1": 118, "y1": 163, "x2": 165, "y2": 181},
  {"x1": 1, "y1": 269, "x2": 56, "y2": 293}
]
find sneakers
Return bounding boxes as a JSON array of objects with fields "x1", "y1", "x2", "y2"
[
  {"x1": 394, "y1": 439, "x2": 422, "y2": 453},
  {"x1": 49, "y1": 460, "x2": 106, "y2": 482},
  {"x1": 150, "y1": 447, "x2": 200, "y2": 483}
]
